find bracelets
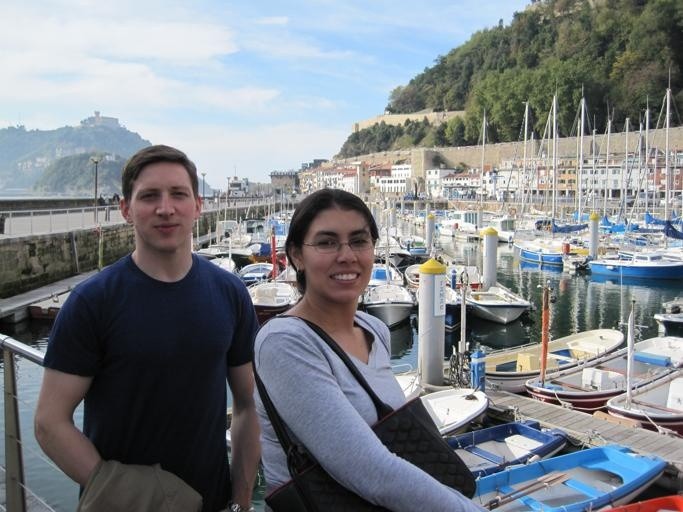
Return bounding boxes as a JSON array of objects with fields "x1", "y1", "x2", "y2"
[{"x1": 226, "y1": 500, "x2": 256, "y2": 512}]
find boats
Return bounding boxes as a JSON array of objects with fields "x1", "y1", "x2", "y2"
[
  {"x1": 466, "y1": 273, "x2": 683, "y2": 427},
  {"x1": 395, "y1": 373, "x2": 683, "y2": 508}
]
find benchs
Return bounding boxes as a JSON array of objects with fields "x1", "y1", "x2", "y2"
[
  {"x1": 472, "y1": 292, "x2": 495, "y2": 296},
  {"x1": 495, "y1": 431, "x2": 547, "y2": 451},
  {"x1": 597, "y1": 365, "x2": 651, "y2": 379},
  {"x1": 552, "y1": 380, "x2": 593, "y2": 392},
  {"x1": 563, "y1": 477, "x2": 607, "y2": 499},
  {"x1": 496, "y1": 484, "x2": 551, "y2": 511},
  {"x1": 633, "y1": 399, "x2": 683, "y2": 415},
  {"x1": 466, "y1": 445, "x2": 508, "y2": 467},
  {"x1": 550, "y1": 353, "x2": 578, "y2": 363},
  {"x1": 634, "y1": 351, "x2": 671, "y2": 368}
]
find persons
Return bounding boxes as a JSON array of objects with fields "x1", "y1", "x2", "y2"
[
  {"x1": 34, "y1": 144, "x2": 260, "y2": 512},
  {"x1": 247, "y1": 188, "x2": 493, "y2": 512}
]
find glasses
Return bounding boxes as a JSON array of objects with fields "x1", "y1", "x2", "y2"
[{"x1": 297, "y1": 234, "x2": 379, "y2": 255}]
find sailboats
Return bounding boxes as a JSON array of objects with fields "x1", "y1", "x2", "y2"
[{"x1": 510, "y1": 87, "x2": 683, "y2": 284}]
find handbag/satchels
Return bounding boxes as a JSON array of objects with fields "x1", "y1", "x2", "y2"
[{"x1": 263, "y1": 396, "x2": 476, "y2": 512}]
